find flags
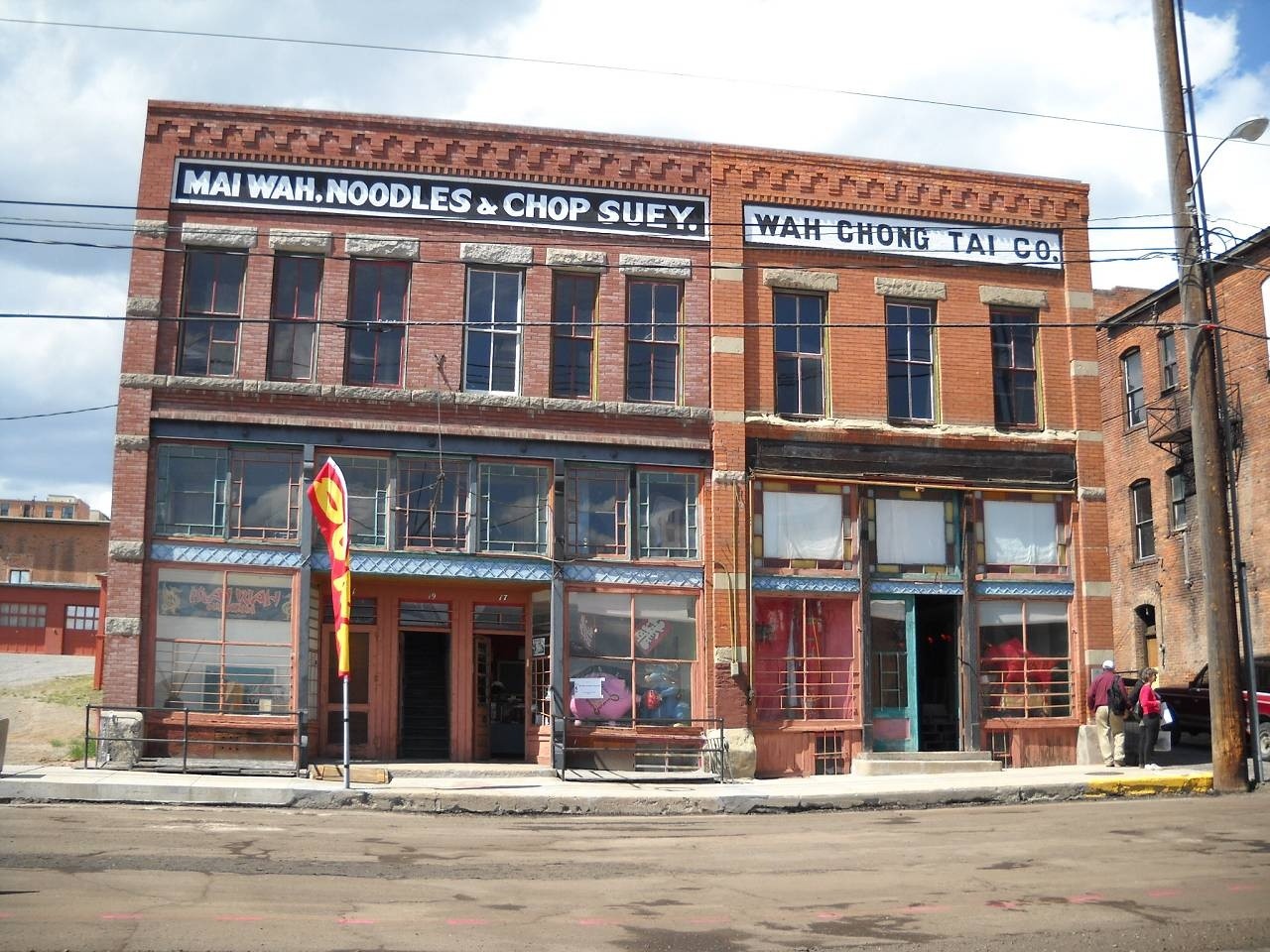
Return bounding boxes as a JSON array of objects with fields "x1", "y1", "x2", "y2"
[{"x1": 304, "y1": 455, "x2": 353, "y2": 676}]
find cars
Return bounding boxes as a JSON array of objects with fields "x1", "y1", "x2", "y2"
[
  {"x1": 1152, "y1": 653, "x2": 1270, "y2": 762},
  {"x1": 1120, "y1": 674, "x2": 1142, "y2": 721}
]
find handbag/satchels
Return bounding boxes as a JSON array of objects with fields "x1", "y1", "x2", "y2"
[
  {"x1": 1153, "y1": 730, "x2": 1172, "y2": 752},
  {"x1": 1106, "y1": 676, "x2": 1125, "y2": 715},
  {"x1": 1133, "y1": 701, "x2": 1143, "y2": 723},
  {"x1": 1159, "y1": 701, "x2": 1173, "y2": 726}
]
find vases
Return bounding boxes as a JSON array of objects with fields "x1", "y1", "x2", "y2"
[{"x1": 165, "y1": 695, "x2": 184, "y2": 708}]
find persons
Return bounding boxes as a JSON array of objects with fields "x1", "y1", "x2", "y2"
[
  {"x1": 1128, "y1": 666, "x2": 1164, "y2": 770},
  {"x1": 1085, "y1": 660, "x2": 1128, "y2": 767}
]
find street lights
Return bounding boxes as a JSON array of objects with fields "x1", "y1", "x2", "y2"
[{"x1": 1193, "y1": 114, "x2": 1270, "y2": 795}]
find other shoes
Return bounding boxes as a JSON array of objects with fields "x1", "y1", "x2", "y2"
[
  {"x1": 1139, "y1": 763, "x2": 1163, "y2": 769},
  {"x1": 1114, "y1": 764, "x2": 1122, "y2": 767}
]
